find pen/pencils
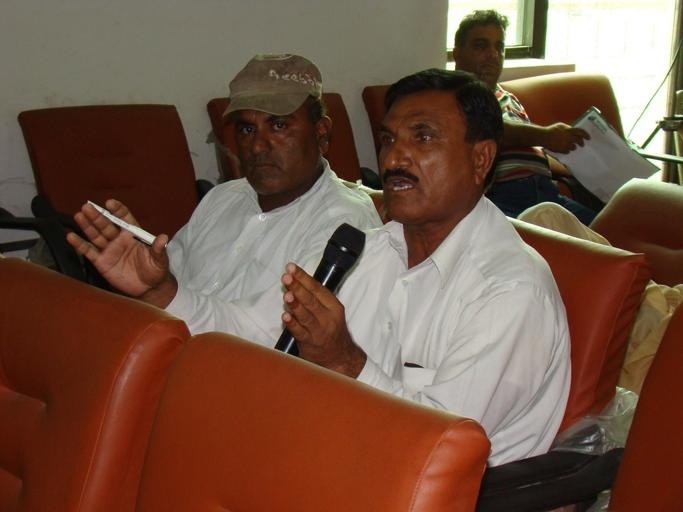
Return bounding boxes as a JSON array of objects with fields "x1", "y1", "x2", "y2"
[{"x1": 86, "y1": 200, "x2": 168, "y2": 249}]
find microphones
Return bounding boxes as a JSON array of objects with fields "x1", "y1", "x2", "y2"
[{"x1": 274, "y1": 224, "x2": 366, "y2": 354}]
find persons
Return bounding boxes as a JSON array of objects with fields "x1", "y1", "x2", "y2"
[
  {"x1": 277, "y1": 66, "x2": 572, "y2": 472},
  {"x1": 452, "y1": 8, "x2": 597, "y2": 234},
  {"x1": 66, "y1": 51, "x2": 384, "y2": 352}
]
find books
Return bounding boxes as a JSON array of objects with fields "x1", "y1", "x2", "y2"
[{"x1": 541, "y1": 104, "x2": 661, "y2": 204}]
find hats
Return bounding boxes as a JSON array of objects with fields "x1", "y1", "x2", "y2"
[{"x1": 219, "y1": 53, "x2": 324, "y2": 118}]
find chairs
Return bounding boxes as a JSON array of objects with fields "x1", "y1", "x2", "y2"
[{"x1": 0, "y1": 72, "x2": 683, "y2": 512}]
